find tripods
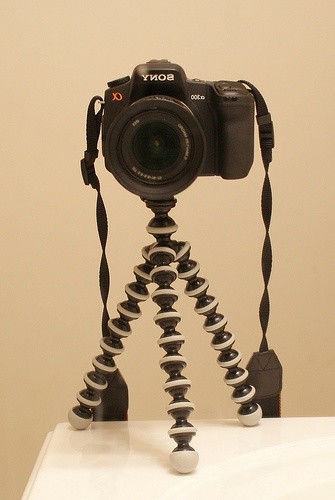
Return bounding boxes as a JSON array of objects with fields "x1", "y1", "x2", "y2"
[{"x1": 67, "y1": 201, "x2": 261, "y2": 475}]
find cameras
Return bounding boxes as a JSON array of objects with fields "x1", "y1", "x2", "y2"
[{"x1": 98, "y1": 57, "x2": 259, "y2": 199}]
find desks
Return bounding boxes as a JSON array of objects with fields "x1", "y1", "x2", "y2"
[{"x1": 22, "y1": 416, "x2": 335, "y2": 500}]
